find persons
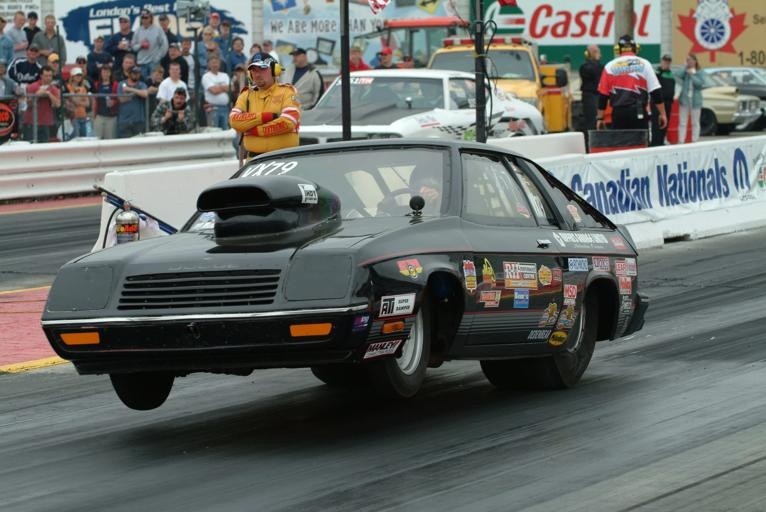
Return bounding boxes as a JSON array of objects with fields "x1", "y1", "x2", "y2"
[
  {"x1": 288, "y1": 47, "x2": 325, "y2": 110},
  {"x1": 408, "y1": 162, "x2": 443, "y2": 215},
  {"x1": 1, "y1": 8, "x2": 280, "y2": 144},
  {"x1": 594, "y1": 34, "x2": 668, "y2": 130},
  {"x1": 414, "y1": 80, "x2": 457, "y2": 110},
  {"x1": 579, "y1": 44, "x2": 603, "y2": 129},
  {"x1": 674, "y1": 52, "x2": 706, "y2": 144},
  {"x1": 339, "y1": 45, "x2": 399, "y2": 72},
  {"x1": 648, "y1": 54, "x2": 675, "y2": 146},
  {"x1": 228, "y1": 51, "x2": 300, "y2": 162}
]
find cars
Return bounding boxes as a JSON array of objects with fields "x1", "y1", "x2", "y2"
[
  {"x1": 296, "y1": 67, "x2": 548, "y2": 145},
  {"x1": 570, "y1": 62, "x2": 765, "y2": 137},
  {"x1": 41, "y1": 137, "x2": 648, "y2": 412}
]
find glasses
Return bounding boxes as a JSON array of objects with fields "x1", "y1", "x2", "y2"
[
  {"x1": 78, "y1": 61, "x2": 85, "y2": 64},
  {"x1": 30, "y1": 49, "x2": 37, "y2": 52}
]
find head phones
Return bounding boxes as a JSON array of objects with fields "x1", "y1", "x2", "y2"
[
  {"x1": 247, "y1": 53, "x2": 283, "y2": 82},
  {"x1": 614, "y1": 40, "x2": 640, "y2": 52},
  {"x1": 584, "y1": 51, "x2": 589, "y2": 59}
]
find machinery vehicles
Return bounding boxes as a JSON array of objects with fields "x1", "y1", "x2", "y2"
[{"x1": 351, "y1": 17, "x2": 469, "y2": 66}]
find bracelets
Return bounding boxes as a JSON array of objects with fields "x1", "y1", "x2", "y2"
[{"x1": 595, "y1": 115, "x2": 603, "y2": 120}]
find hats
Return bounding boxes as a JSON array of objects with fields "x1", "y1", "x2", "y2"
[
  {"x1": 247, "y1": 52, "x2": 275, "y2": 71},
  {"x1": 262, "y1": 40, "x2": 271, "y2": 46},
  {"x1": 0, "y1": 9, "x2": 179, "y2": 78},
  {"x1": 289, "y1": 48, "x2": 306, "y2": 55},
  {"x1": 203, "y1": 12, "x2": 245, "y2": 70},
  {"x1": 618, "y1": 34, "x2": 635, "y2": 48},
  {"x1": 379, "y1": 47, "x2": 392, "y2": 56}
]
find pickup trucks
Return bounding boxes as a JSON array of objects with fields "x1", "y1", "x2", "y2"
[{"x1": 425, "y1": 36, "x2": 572, "y2": 134}]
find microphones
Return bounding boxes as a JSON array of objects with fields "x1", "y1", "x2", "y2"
[{"x1": 248, "y1": 86, "x2": 259, "y2": 91}]
